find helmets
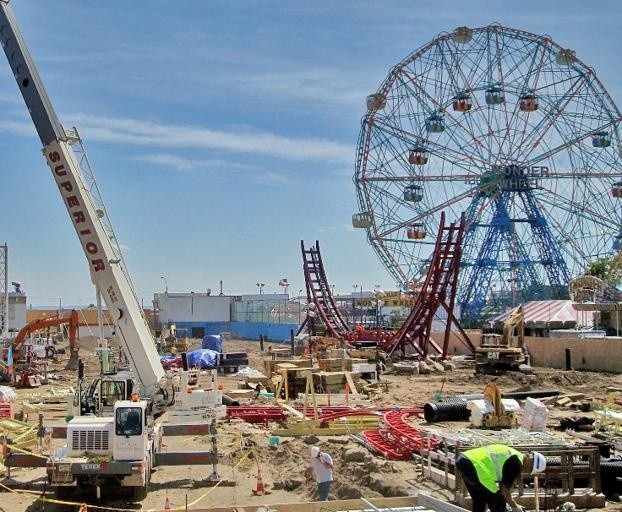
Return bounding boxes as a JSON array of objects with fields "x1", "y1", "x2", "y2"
[
  {"x1": 530, "y1": 450, "x2": 546, "y2": 475},
  {"x1": 310, "y1": 446, "x2": 319, "y2": 458}
]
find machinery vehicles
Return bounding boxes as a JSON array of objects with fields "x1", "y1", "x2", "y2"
[
  {"x1": 476, "y1": 304, "x2": 532, "y2": 375},
  {"x1": 0, "y1": 310, "x2": 79, "y2": 383},
  {"x1": 1, "y1": 0, "x2": 225, "y2": 501}
]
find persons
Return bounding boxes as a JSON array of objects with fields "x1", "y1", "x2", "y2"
[
  {"x1": 455, "y1": 444, "x2": 547, "y2": 512},
  {"x1": 311, "y1": 446, "x2": 334, "y2": 501}
]
